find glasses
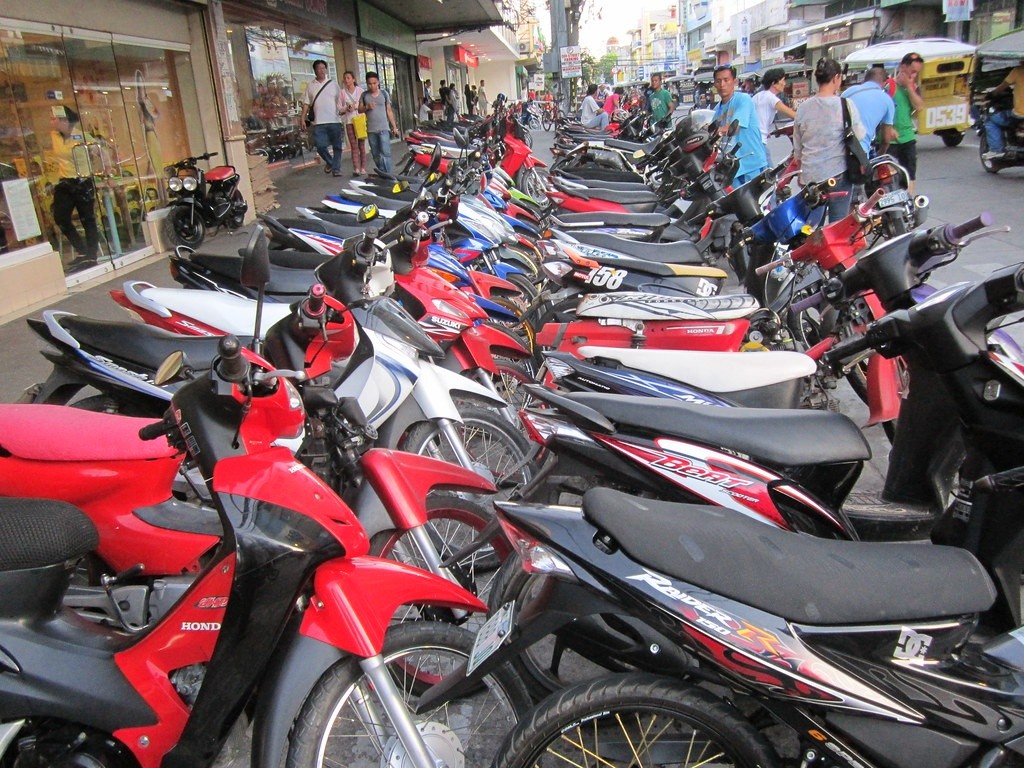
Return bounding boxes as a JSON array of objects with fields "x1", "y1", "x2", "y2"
[{"x1": 714, "y1": 64, "x2": 731, "y2": 71}]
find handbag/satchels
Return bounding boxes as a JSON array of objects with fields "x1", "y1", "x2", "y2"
[
  {"x1": 305, "y1": 107, "x2": 314, "y2": 121},
  {"x1": 841, "y1": 98, "x2": 873, "y2": 184},
  {"x1": 352, "y1": 111, "x2": 368, "y2": 140}
]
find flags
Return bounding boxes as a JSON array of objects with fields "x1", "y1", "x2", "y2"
[
  {"x1": 946, "y1": 0, "x2": 970, "y2": 21},
  {"x1": 736, "y1": 11, "x2": 750, "y2": 56}
]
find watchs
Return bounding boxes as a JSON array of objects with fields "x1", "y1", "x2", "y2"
[{"x1": 107, "y1": 174, "x2": 113, "y2": 177}]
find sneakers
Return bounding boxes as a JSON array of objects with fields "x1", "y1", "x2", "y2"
[
  {"x1": 981, "y1": 151, "x2": 1006, "y2": 160},
  {"x1": 64, "y1": 255, "x2": 97, "y2": 272}
]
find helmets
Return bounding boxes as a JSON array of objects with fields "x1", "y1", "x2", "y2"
[
  {"x1": 610, "y1": 110, "x2": 629, "y2": 122},
  {"x1": 497, "y1": 93, "x2": 505, "y2": 101}
]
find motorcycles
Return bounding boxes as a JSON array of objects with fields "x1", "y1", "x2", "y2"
[
  {"x1": 415, "y1": 106, "x2": 1024, "y2": 768},
  {"x1": 837, "y1": 38, "x2": 975, "y2": 146},
  {"x1": 610, "y1": 82, "x2": 647, "y2": 94},
  {"x1": 739, "y1": 63, "x2": 813, "y2": 130},
  {"x1": 0, "y1": 102, "x2": 549, "y2": 768},
  {"x1": 964, "y1": 27, "x2": 1024, "y2": 172},
  {"x1": 692, "y1": 72, "x2": 720, "y2": 110},
  {"x1": 662, "y1": 76, "x2": 696, "y2": 103}
]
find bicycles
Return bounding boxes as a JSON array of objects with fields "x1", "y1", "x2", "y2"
[{"x1": 542, "y1": 96, "x2": 565, "y2": 132}]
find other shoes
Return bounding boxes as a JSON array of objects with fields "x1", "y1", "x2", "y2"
[
  {"x1": 324, "y1": 164, "x2": 332, "y2": 173},
  {"x1": 332, "y1": 170, "x2": 342, "y2": 177},
  {"x1": 352, "y1": 169, "x2": 366, "y2": 177}
]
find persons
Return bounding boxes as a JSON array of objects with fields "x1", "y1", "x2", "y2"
[
  {"x1": 464, "y1": 80, "x2": 489, "y2": 116},
  {"x1": 419, "y1": 78, "x2": 461, "y2": 123},
  {"x1": 792, "y1": 52, "x2": 866, "y2": 228},
  {"x1": 358, "y1": 72, "x2": 399, "y2": 174},
  {"x1": 580, "y1": 73, "x2": 675, "y2": 131},
  {"x1": 980, "y1": 59, "x2": 1024, "y2": 160},
  {"x1": 522, "y1": 86, "x2": 554, "y2": 114},
  {"x1": 884, "y1": 52, "x2": 925, "y2": 198},
  {"x1": 48, "y1": 104, "x2": 118, "y2": 275},
  {"x1": 839, "y1": 67, "x2": 895, "y2": 215},
  {"x1": 135, "y1": 70, "x2": 170, "y2": 209},
  {"x1": 300, "y1": 59, "x2": 342, "y2": 177},
  {"x1": 336, "y1": 71, "x2": 367, "y2": 177},
  {"x1": 712, "y1": 65, "x2": 796, "y2": 189}
]
